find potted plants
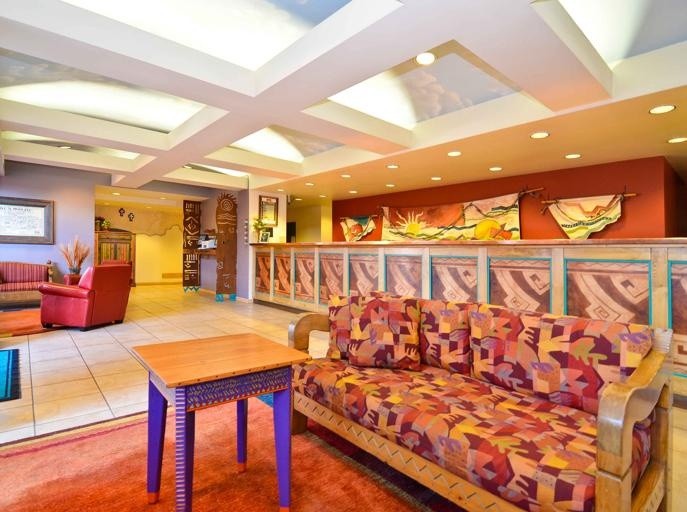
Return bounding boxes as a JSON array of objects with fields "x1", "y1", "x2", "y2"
[{"x1": 101, "y1": 218, "x2": 112, "y2": 231}]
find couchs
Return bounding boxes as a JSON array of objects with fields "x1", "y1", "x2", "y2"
[
  {"x1": 288, "y1": 312, "x2": 675, "y2": 511},
  {"x1": 0, "y1": 260, "x2": 54, "y2": 304}
]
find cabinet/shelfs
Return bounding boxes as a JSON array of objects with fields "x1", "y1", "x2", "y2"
[{"x1": 95, "y1": 228, "x2": 136, "y2": 287}]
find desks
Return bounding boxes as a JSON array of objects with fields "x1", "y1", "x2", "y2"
[
  {"x1": 64, "y1": 274, "x2": 81, "y2": 285},
  {"x1": 130, "y1": 333, "x2": 314, "y2": 512}
]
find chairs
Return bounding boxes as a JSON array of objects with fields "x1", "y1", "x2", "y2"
[{"x1": 38, "y1": 260, "x2": 132, "y2": 331}]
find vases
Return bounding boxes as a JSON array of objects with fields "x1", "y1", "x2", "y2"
[{"x1": 70, "y1": 268, "x2": 80, "y2": 275}]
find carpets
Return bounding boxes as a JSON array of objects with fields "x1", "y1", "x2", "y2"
[
  {"x1": 0, "y1": 304, "x2": 65, "y2": 338},
  {"x1": 0, "y1": 349, "x2": 19, "y2": 401},
  {"x1": 0, "y1": 391, "x2": 469, "y2": 512}
]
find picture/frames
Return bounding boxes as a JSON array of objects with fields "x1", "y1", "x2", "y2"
[
  {"x1": 0, "y1": 196, "x2": 54, "y2": 246},
  {"x1": 259, "y1": 195, "x2": 278, "y2": 226},
  {"x1": 258, "y1": 232, "x2": 270, "y2": 243}
]
